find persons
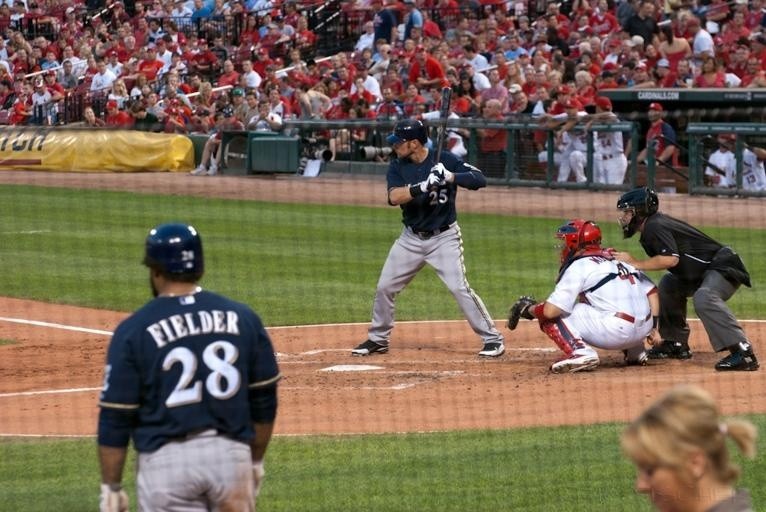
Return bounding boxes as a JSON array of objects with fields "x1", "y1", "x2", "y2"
[
  {"x1": 615, "y1": 386, "x2": 760, "y2": 512},
  {"x1": 350, "y1": 118, "x2": 505, "y2": 356},
  {"x1": 95, "y1": 226, "x2": 281, "y2": 512},
  {"x1": 1, "y1": 1, "x2": 766, "y2": 199},
  {"x1": 605, "y1": 187, "x2": 760, "y2": 371},
  {"x1": 508, "y1": 220, "x2": 661, "y2": 369}
]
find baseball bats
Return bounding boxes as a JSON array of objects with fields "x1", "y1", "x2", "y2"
[{"x1": 430, "y1": 86, "x2": 452, "y2": 191}]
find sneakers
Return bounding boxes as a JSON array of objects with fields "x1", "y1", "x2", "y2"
[
  {"x1": 715, "y1": 352, "x2": 760, "y2": 372},
  {"x1": 351, "y1": 338, "x2": 389, "y2": 357},
  {"x1": 647, "y1": 341, "x2": 692, "y2": 360},
  {"x1": 623, "y1": 352, "x2": 650, "y2": 368},
  {"x1": 190, "y1": 164, "x2": 218, "y2": 175},
  {"x1": 549, "y1": 352, "x2": 599, "y2": 373},
  {"x1": 478, "y1": 341, "x2": 506, "y2": 358}
]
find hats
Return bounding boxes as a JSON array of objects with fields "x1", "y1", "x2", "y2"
[{"x1": 0, "y1": 0, "x2": 766, "y2": 140}]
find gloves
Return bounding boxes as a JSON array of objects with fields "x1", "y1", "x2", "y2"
[
  {"x1": 421, "y1": 162, "x2": 450, "y2": 194},
  {"x1": 251, "y1": 461, "x2": 264, "y2": 500},
  {"x1": 98, "y1": 483, "x2": 128, "y2": 512}
]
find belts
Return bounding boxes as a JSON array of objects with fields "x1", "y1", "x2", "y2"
[{"x1": 404, "y1": 223, "x2": 449, "y2": 238}]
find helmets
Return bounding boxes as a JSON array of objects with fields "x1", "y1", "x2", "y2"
[
  {"x1": 141, "y1": 222, "x2": 202, "y2": 279},
  {"x1": 392, "y1": 119, "x2": 429, "y2": 146},
  {"x1": 616, "y1": 187, "x2": 659, "y2": 239},
  {"x1": 553, "y1": 218, "x2": 602, "y2": 262}
]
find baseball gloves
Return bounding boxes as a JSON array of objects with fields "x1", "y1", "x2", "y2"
[{"x1": 507, "y1": 295, "x2": 536, "y2": 330}]
